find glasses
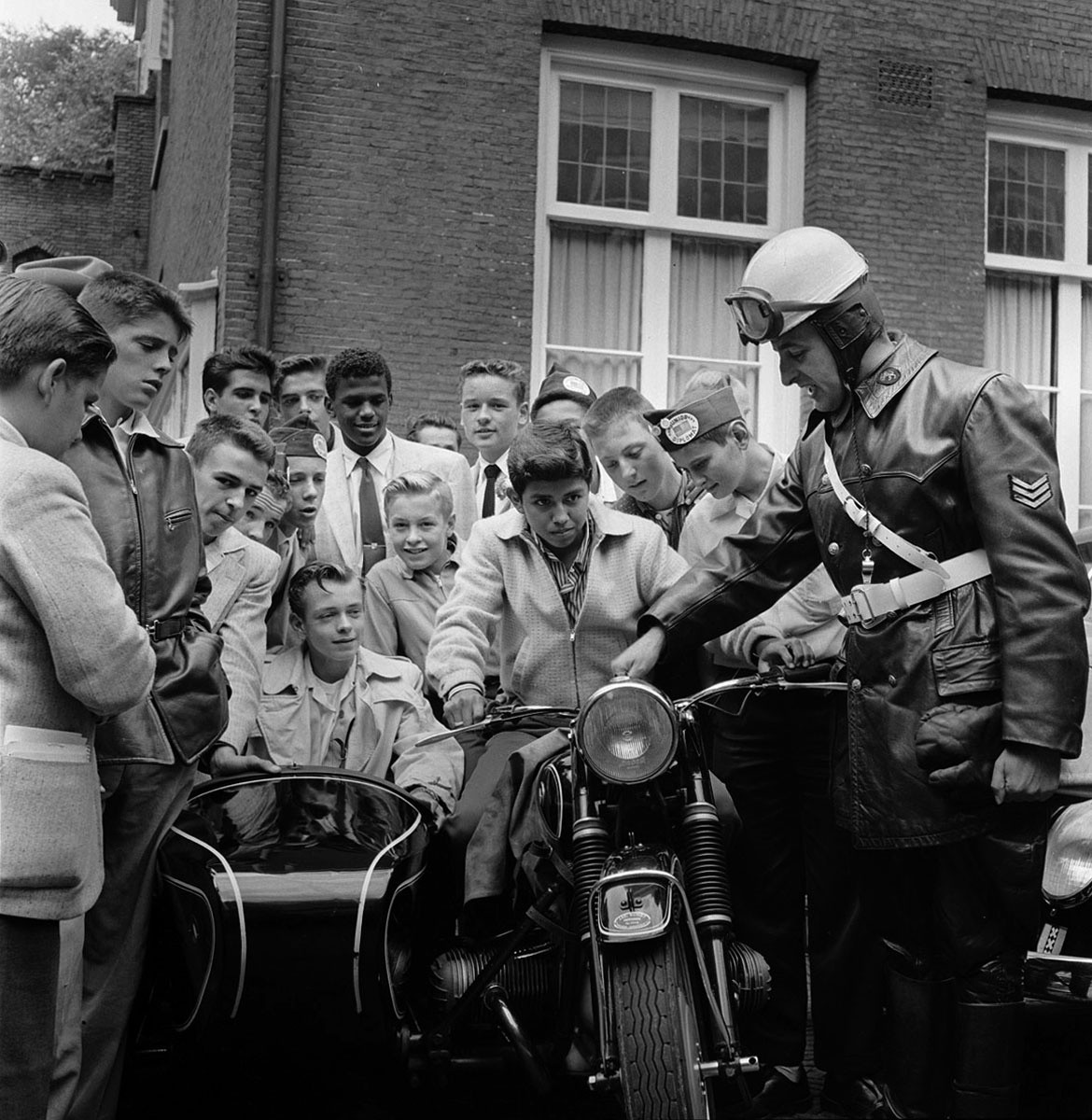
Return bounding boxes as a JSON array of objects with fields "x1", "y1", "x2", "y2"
[{"x1": 723, "y1": 286, "x2": 843, "y2": 348}]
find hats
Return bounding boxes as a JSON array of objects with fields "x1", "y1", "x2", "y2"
[
  {"x1": 531, "y1": 361, "x2": 597, "y2": 422},
  {"x1": 641, "y1": 368, "x2": 743, "y2": 451},
  {"x1": 268, "y1": 413, "x2": 327, "y2": 463},
  {"x1": 14, "y1": 256, "x2": 114, "y2": 298}
]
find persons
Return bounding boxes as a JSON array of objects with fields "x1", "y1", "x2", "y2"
[
  {"x1": 1, "y1": 273, "x2": 156, "y2": 1120},
  {"x1": 60, "y1": 273, "x2": 232, "y2": 1120},
  {"x1": 187, "y1": 337, "x2": 846, "y2": 1120},
  {"x1": 611, "y1": 226, "x2": 1092, "y2": 1120}
]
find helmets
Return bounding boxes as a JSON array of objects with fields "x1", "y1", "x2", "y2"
[{"x1": 724, "y1": 226, "x2": 870, "y2": 344}]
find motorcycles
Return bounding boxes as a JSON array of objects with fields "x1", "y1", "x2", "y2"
[{"x1": 138, "y1": 654, "x2": 1092, "y2": 1120}]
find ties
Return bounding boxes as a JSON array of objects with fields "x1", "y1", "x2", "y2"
[
  {"x1": 356, "y1": 457, "x2": 387, "y2": 582},
  {"x1": 482, "y1": 463, "x2": 501, "y2": 525}
]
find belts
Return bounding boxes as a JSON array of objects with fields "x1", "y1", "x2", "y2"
[
  {"x1": 837, "y1": 550, "x2": 991, "y2": 635},
  {"x1": 137, "y1": 617, "x2": 191, "y2": 645}
]
